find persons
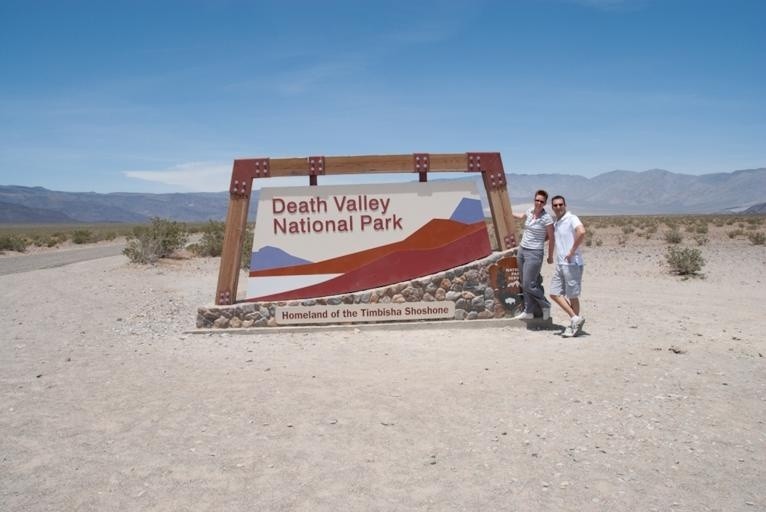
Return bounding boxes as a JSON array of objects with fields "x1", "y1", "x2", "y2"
[
  {"x1": 511, "y1": 190, "x2": 555, "y2": 321},
  {"x1": 545, "y1": 195, "x2": 586, "y2": 337}
]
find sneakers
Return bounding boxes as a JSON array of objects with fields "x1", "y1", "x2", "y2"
[
  {"x1": 541, "y1": 307, "x2": 550, "y2": 320},
  {"x1": 514, "y1": 311, "x2": 534, "y2": 320},
  {"x1": 561, "y1": 315, "x2": 586, "y2": 337}
]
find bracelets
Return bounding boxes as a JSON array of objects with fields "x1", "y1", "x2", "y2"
[{"x1": 569, "y1": 251, "x2": 574, "y2": 256}]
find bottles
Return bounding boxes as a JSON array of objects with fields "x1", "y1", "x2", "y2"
[{"x1": 557, "y1": 255, "x2": 579, "y2": 265}]
